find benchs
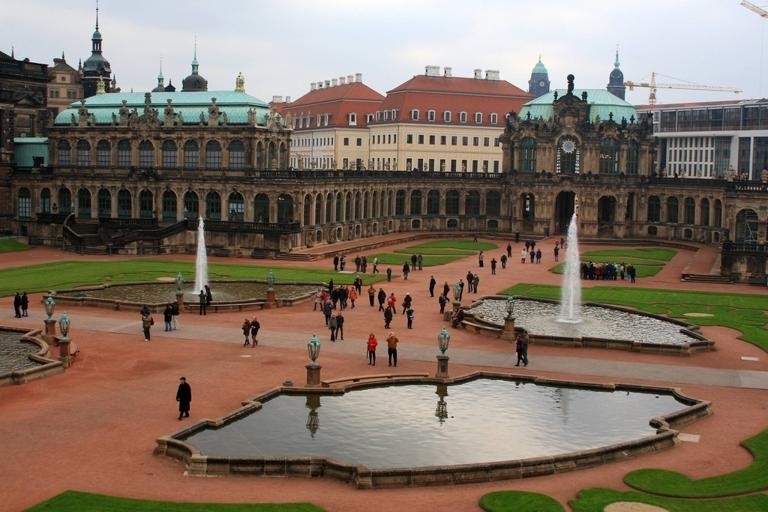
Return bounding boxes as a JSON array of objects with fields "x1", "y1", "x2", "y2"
[
  {"x1": 70, "y1": 340, "x2": 79, "y2": 360},
  {"x1": 317, "y1": 372, "x2": 430, "y2": 387},
  {"x1": 459, "y1": 318, "x2": 502, "y2": 339},
  {"x1": 187, "y1": 302, "x2": 265, "y2": 313}
]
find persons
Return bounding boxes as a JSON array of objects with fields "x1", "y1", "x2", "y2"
[
  {"x1": 175, "y1": 376, "x2": 191, "y2": 421},
  {"x1": 21, "y1": 291, "x2": 29, "y2": 317},
  {"x1": 579, "y1": 261, "x2": 637, "y2": 282},
  {"x1": 313, "y1": 228, "x2": 565, "y2": 367},
  {"x1": 204, "y1": 284, "x2": 213, "y2": 306},
  {"x1": 13, "y1": 292, "x2": 21, "y2": 318},
  {"x1": 198, "y1": 290, "x2": 207, "y2": 315},
  {"x1": 140, "y1": 301, "x2": 179, "y2": 342},
  {"x1": 249, "y1": 316, "x2": 261, "y2": 347},
  {"x1": 241, "y1": 318, "x2": 251, "y2": 347}
]
